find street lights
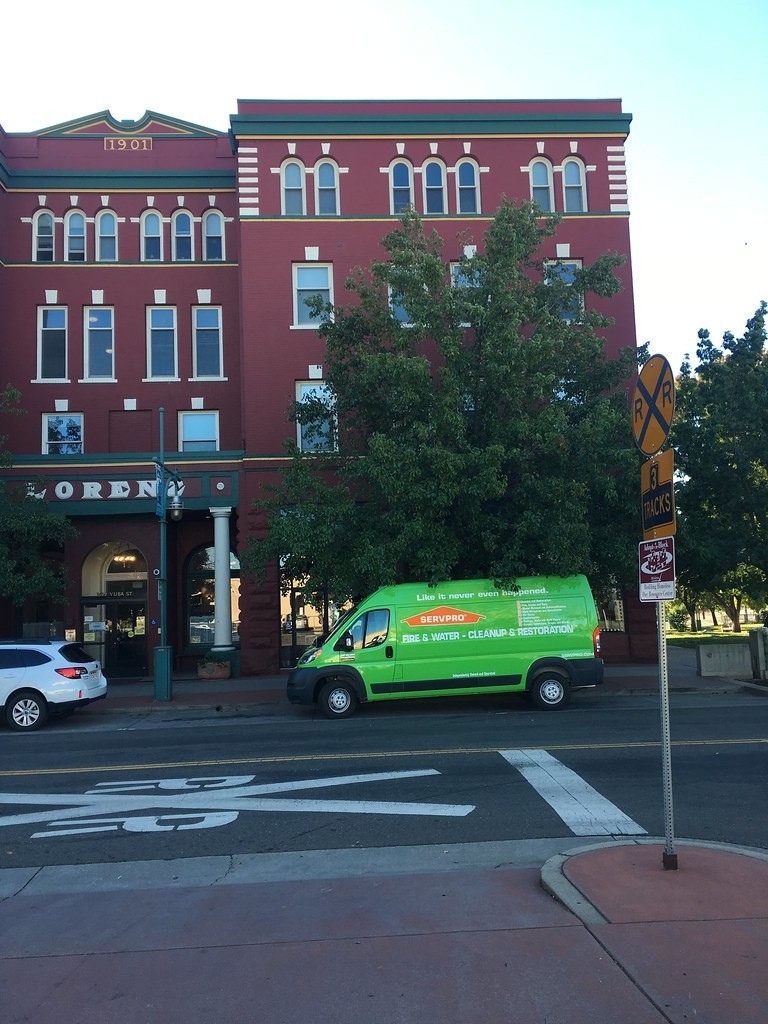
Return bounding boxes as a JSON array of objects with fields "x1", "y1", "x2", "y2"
[{"x1": 151, "y1": 456, "x2": 185, "y2": 701}]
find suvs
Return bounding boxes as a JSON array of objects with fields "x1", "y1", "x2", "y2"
[{"x1": 0, "y1": 636, "x2": 109, "y2": 732}]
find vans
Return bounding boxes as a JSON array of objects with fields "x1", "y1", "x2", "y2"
[{"x1": 286, "y1": 576, "x2": 605, "y2": 720}]
